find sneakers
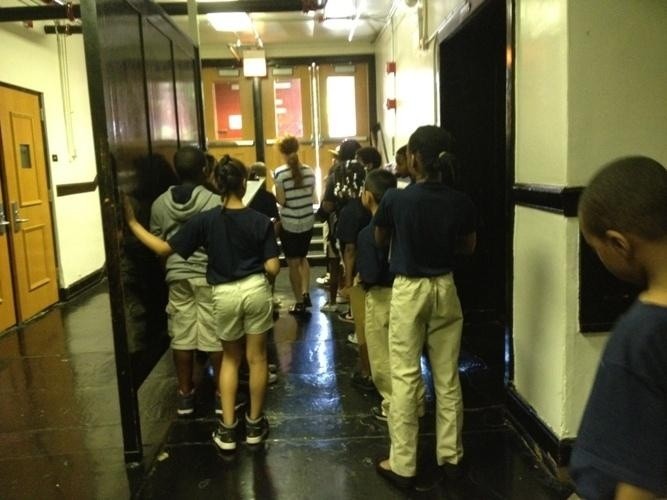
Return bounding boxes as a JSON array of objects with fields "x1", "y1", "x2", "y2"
[
  {"x1": 210, "y1": 416, "x2": 239, "y2": 451},
  {"x1": 287, "y1": 293, "x2": 313, "y2": 315},
  {"x1": 175, "y1": 380, "x2": 198, "y2": 414},
  {"x1": 347, "y1": 330, "x2": 359, "y2": 344},
  {"x1": 316, "y1": 272, "x2": 331, "y2": 289},
  {"x1": 374, "y1": 456, "x2": 416, "y2": 488},
  {"x1": 337, "y1": 311, "x2": 355, "y2": 323},
  {"x1": 213, "y1": 389, "x2": 249, "y2": 414},
  {"x1": 349, "y1": 372, "x2": 376, "y2": 391},
  {"x1": 370, "y1": 406, "x2": 389, "y2": 422},
  {"x1": 244, "y1": 409, "x2": 270, "y2": 446},
  {"x1": 319, "y1": 301, "x2": 338, "y2": 313},
  {"x1": 239, "y1": 360, "x2": 279, "y2": 387}
]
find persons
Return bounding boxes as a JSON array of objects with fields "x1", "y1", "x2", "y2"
[{"x1": 567, "y1": 156, "x2": 667, "y2": 500}]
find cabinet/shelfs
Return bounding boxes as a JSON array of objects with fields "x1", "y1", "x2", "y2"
[{"x1": 305, "y1": 203, "x2": 328, "y2": 259}]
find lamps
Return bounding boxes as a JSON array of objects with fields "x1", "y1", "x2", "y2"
[
  {"x1": 242, "y1": 50, "x2": 267, "y2": 77},
  {"x1": 210, "y1": 12, "x2": 258, "y2": 33}
]
